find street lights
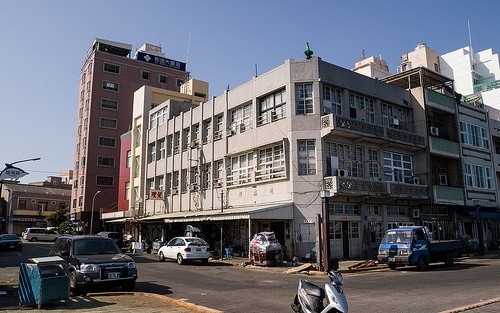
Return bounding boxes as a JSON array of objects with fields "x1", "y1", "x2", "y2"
[
  {"x1": 91, "y1": 191, "x2": 102, "y2": 234},
  {"x1": 0, "y1": 158, "x2": 40, "y2": 175}
]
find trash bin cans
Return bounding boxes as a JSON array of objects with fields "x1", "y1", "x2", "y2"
[{"x1": 17, "y1": 256, "x2": 70, "y2": 310}]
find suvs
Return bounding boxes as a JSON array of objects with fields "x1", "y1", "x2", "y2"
[{"x1": 49, "y1": 234, "x2": 137, "y2": 295}]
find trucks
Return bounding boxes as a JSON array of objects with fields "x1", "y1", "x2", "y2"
[{"x1": 378, "y1": 227, "x2": 462, "y2": 270}]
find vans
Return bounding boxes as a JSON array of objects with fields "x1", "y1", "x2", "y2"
[
  {"x1": 23, "y1": 228, "x2": 59, "y2": 241},
  {"x1": 96, "y1": 232, "x2": 122, "y2": 249}
]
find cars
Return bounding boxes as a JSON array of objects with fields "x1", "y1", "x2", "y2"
[
  {"x1": 158, "y1": 237, "x2": 209, "y2": 264},
  {"x1": 0, "y1": 234, "x2": 22, "y2": 251}
]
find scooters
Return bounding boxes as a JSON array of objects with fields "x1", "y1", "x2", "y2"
[{"x1": 292, "y1": 268, "x2": 348, "y2": 313}]
